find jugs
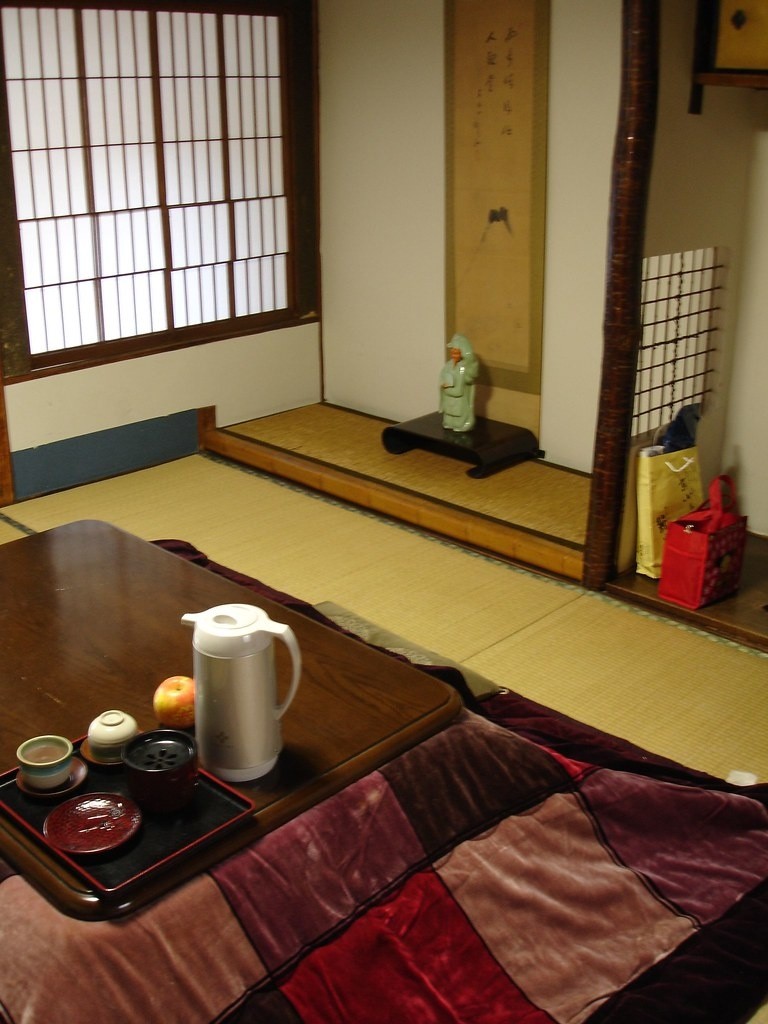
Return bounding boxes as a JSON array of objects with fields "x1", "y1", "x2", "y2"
[{"x1": 179, "y1": 602, "x2": 302, "y2": 783}]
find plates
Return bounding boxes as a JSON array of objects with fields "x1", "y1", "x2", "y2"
[
  {"x1": 43, "y1": 792, "x2": 142, "y2": 854},
  {"x1": 79, "y1": 729, "x2": 144, "y2": 763},
  {"x1": 0, "y1": 730, "x2": 255, "y2": 895},
  {"x1": 17, "y1": 758, "x2": 88, "y2": 796}
]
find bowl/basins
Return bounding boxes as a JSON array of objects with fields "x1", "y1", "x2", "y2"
[
  {"x1": 86, "y1": 710, "x2": 138, "y2": 763},
  {"x1": 17, "y1": 735, "x2": 73, "y2": 789}
]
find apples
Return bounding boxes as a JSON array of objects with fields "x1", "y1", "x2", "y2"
[{"x1": 153, "y1": 674, "x2": 196, "y2": 730}]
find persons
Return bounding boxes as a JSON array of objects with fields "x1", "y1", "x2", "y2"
[{"x1": 438, "y1": 335, "x2": 478, "y2": 432}]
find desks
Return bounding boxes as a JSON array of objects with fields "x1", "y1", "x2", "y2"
[{"x1": 0, "y1": 519, "x2": 461, "y2": 923}]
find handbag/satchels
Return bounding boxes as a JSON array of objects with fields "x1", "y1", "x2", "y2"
[
  {"x1": 658, "y1": 475, "x2": 748, "y2": 610},
  {"x1": 635, "y1": 422, "x2": 706, "y2": 579}
]
